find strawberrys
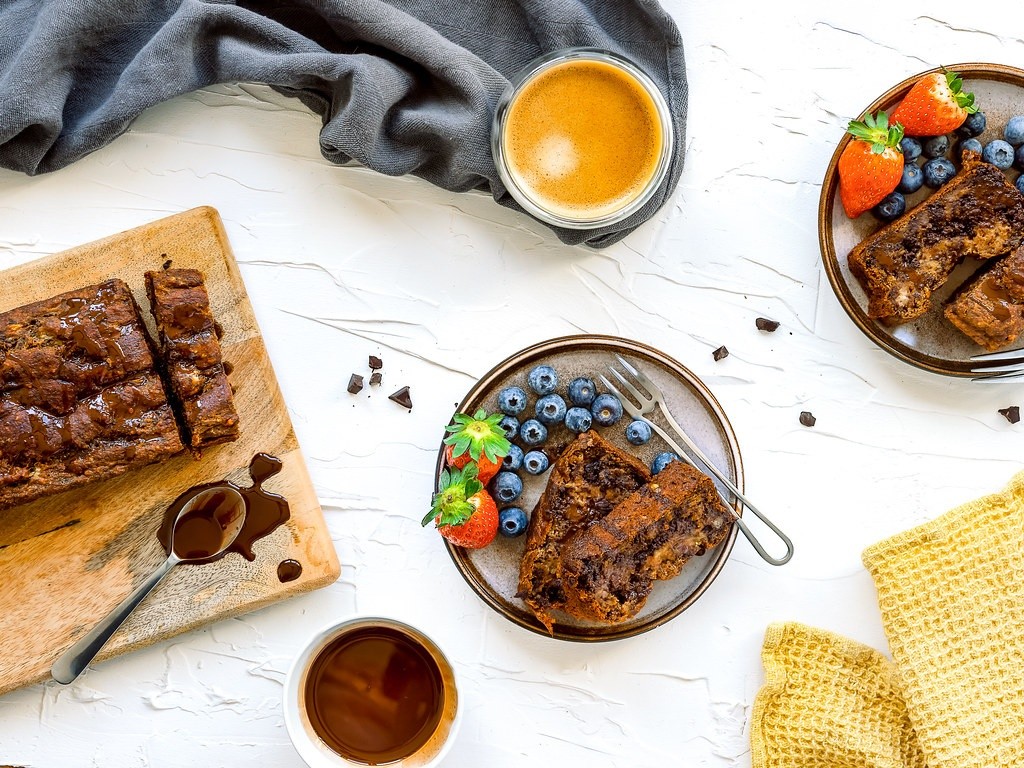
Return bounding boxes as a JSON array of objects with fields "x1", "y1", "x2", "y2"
[
  {"x1": 888, "y1": 65, "x2": 981, "y2": 137},
  {"x1": 421, "y1": 459, "x2": 499, "y2": 549},
  {"x1": 838, "y1": 109, "x2": 905, "y2": 220},
  {"x1": 441, "y1": 407, "x2": 512, "y2": 487}
]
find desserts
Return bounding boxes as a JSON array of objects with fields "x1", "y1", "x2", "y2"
[
  {"x1": 847, "y1": 148, "x2": 1024, "y2": 351},
  {"x1": 513, "y1": 428, "x2": 738, "y2": 637},
  {"x1": 0, "y1": 268, "x2": 239, "y2": 510}
]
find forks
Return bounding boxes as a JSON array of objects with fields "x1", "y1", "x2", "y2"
[
  {"x1": 969, "y1": 349, "x2": 1023, "y2": 386},
  {"x1": 595, "y1": 347, "x2": 794, "y2": 569}
]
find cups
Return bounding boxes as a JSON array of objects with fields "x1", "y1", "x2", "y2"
[
  {"x1": 488, "y1": 45, "x2": 680, "y2": 229},
  {"x1": 279, "y1": 616, "x2": 463, "y2": 768}
]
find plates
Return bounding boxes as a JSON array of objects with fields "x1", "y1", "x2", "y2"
[
  {"x1": 813, "y1": 61, "x2": 1024, "y2": 380},
  {"x1": 434, "y1": 333, "x2": 746, "y2": 645}
]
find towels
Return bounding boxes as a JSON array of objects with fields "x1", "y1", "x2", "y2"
[{"x1": 0, "y1": 0, "x2": 693, "y2": 250}]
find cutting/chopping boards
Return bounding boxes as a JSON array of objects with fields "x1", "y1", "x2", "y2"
[{"x1": 0, "y1": 205, "x2": 344, "y2": 698}]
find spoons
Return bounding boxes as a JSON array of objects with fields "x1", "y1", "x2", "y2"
[{"x1": 47, "y1": 486, "x2": 245, "y2": 685}]
